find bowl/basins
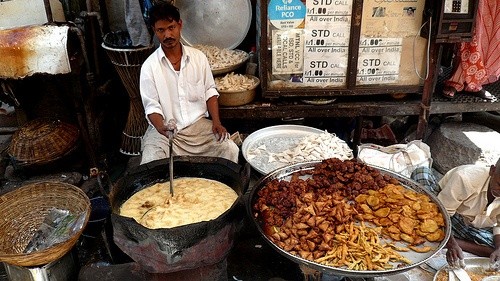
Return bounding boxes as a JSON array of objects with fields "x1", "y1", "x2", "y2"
[
  {"x1": 216, "y1": 75, "x2": 260, "y2": 107},
  {"x1": 213, "y1": 48, "x2": 250, "y2": 75}
]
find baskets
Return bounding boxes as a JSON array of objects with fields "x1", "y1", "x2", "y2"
[{"x1": 0, "y1": 182, "x2": 91, "y2": 266}]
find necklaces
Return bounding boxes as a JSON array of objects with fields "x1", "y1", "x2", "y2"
[{"x1": 171, "y1": 59, "x2": 181, "y2": 65}]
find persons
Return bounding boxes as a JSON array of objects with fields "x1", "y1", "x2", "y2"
[
  {"x1": 140, "y1": 2, "x2": 240, "y2": 167},
  {"x1": 411, "y1": 157, "x2": 500, "y2": 271}
]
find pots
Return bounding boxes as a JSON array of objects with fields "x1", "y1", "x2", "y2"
[{"x1": 100, "y1": 155, "x2": 250, "y2": 273}]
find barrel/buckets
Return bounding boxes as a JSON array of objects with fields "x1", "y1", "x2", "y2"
[
  {"x1": 3, "y1": 252, "x2": 71, "y2": 281},
  {"x1": 79, "y1": 196, "x2": 131, "y2": 265}
]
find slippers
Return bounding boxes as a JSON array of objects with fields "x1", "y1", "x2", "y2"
[{"x1": 441, "y1": 86, "x2": 457, "y2": 99}]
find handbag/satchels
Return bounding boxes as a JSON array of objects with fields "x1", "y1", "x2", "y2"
[{"x1": 358, "y1": 139, "x2": 433, "y2": 180}]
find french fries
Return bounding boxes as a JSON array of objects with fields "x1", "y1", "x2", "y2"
[{"x1": 314, "y1": 220, "x2": 432, "y2": 270}]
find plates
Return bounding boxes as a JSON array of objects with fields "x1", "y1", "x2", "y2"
[
  {"x1": 248, "y1": 160, "x2": 451, "y2": 277},
  {"x1": 242, "y1": 124, "x2": 354, "y2": 176},
  {"x1": 432, "y1": 257, "x2": 500, "y2": 281}
]
